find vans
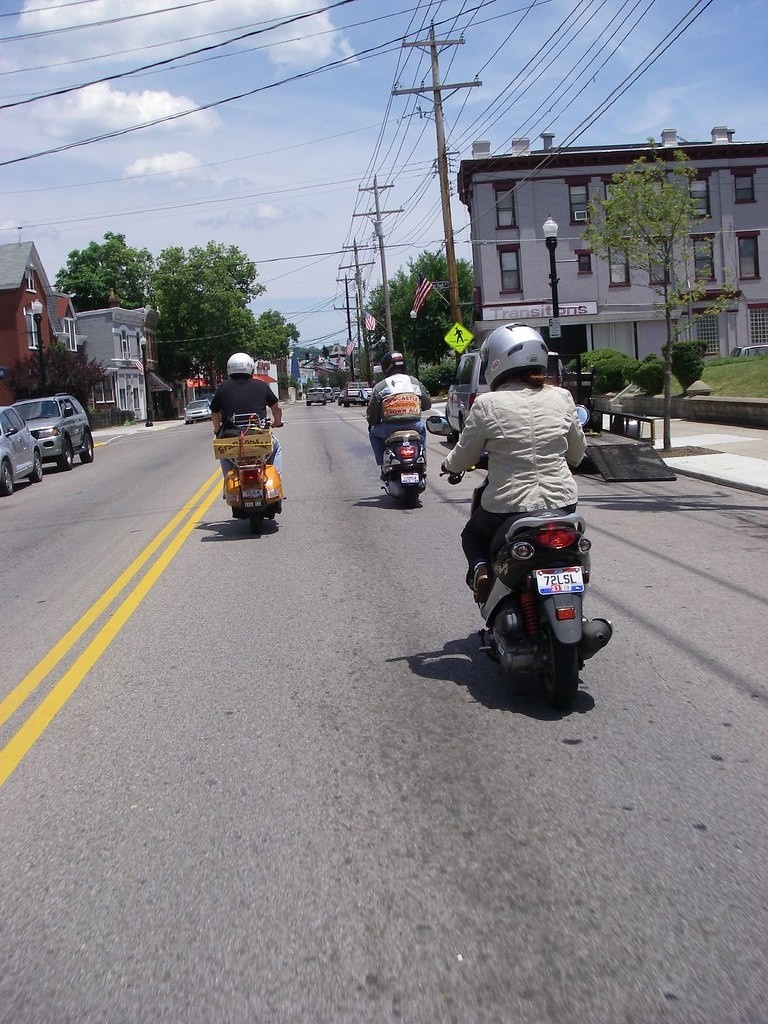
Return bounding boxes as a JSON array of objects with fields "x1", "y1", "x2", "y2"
[{"x1": 444, "y1": 350, "x2": 561, "y2": 444}]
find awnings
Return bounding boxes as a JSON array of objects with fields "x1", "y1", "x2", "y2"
[
  {"x1": 251, "y1": 374, "x2": 277, "y2": 383},
  {"x1": 147, "y1": 370, "x2": 176, "y2": 392},
  {"x1": 186, "y1": 377, "x2": 224, "y2": 387}
]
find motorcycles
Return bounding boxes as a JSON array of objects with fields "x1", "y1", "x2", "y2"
[
  {"x1": 358, "y1": 389, "x2": 427, "y2": 505},
  {"x1": 213, "y1": 413, "x2": 290, "y2": 534},
  {"x1": 427, "y1": 405, "x2": 614, "y2": 704}
]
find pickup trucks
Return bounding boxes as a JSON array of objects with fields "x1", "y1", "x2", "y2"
[
  {"x1": 338, "y1": 381, "x2": 372, "y2": 406},
  {"x1": 305, "y1": 388, "x2": 326, "y2": 406},
  {"x1": 563, "y1": 372, "x2": 593, "y2": 404}
]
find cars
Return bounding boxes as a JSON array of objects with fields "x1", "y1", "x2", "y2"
[
  {"x1": 333, "y1": 387, "x2": 340, "y2": 399},
  {"x1": 730, "y1": 344, "x2": 768, "y2": 357},
  {"x1": 183, "y1": 399, "x2": 212, "y2": 424},
  {"x1": 0, "y1": 406, "x2": 43, "y2": 496}
]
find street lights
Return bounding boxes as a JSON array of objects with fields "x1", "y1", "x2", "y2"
[
  {"x1": 33, "y1": 299, "x2": 48, "y2": 398},
  {"x1": 410, "y1": 311, "x2": 420, "y2": 381},
  {"x1": 543, "y1": 217, "x2": 561, "y2": 383},
  {"x1": 140, "y1": 336, "x2": 153, "y2": 427}
]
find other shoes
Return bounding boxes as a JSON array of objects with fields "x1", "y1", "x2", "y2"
[
  {"x1": 473, "y1": 562, "x2": 489, "y2": 597},
  {"x1": 379, "y1": 465, "x2": 387, "y2": 482}
]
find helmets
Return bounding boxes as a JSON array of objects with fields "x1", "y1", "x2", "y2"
[
  {"x1": 481, "y1": 322, "x2": 549, "y2": 391},
  {"x1": 380, "y1": 350, "x2": 405, "y2": 375},
  {"x1": 227, "y1": 353, "x2": 255, "y2": 377}
]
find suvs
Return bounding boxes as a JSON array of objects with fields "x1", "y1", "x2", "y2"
[
  {"x1": 323, "y1": 387, "x2": 335, "y2": 402},
  {"x1": 8, "y1": 393, "x2": 95, "y2": 471}
]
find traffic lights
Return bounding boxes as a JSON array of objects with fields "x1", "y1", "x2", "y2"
[
  {"x1": 324, "y1": 351, "x2": 329, "y2": 359},
  {"x1": 305, "y1": 352, "x2": 309, "y2": 363}
]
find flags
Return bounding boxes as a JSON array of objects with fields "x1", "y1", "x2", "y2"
[
  {"x1": 344, "y1": 338, "x2": 355, "y2": 355},
  {"x1": 336, "y1": 355, "x2": 345, "y2": 367},
  {"x1": 363, "y1": 310, "x2": 376, "y2": 331},
  {"x1": 411, "y1": 272, "x2": 433, "y2": 318}
]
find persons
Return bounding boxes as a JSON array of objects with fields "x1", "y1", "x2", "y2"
[
  {"x1": 210, "y1": 353, "x2": 288, "y2": 505},
  {"x1": 366, "y1": 351, "x2": 433, "y2": 475},
  {"x1": 440, "y1": 323, "x2": 586, "y2": 603}
]
